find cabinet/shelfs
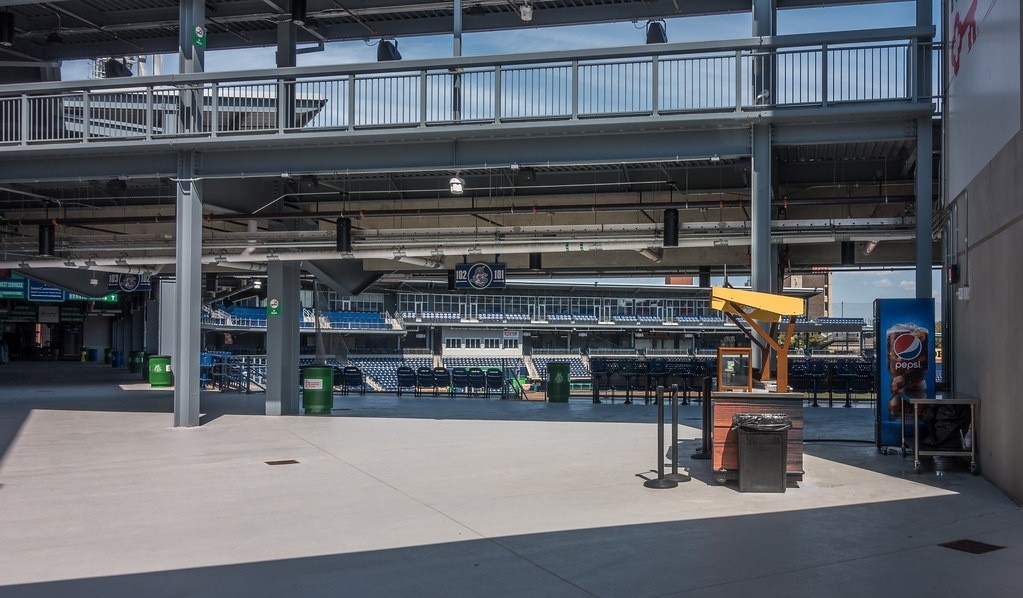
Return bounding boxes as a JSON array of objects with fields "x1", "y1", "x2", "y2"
[{"x1": 708, "y1": 389, "x2": 805, "y2": 475}]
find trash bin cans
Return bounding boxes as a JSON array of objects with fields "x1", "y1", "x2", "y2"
[
  {"x1": 149, "y1": 355, "x2": 172, "y2": 387},
  {"x1": 546, "y1": 361, "x2": 572, "y2": 403},
  {"x1": 128, "y1": 351, "x2": 144, "y2": 373},
  {"x1": 302, "y1": 364, "x2": 335, "y2": 415},
  {"x1": 88, "y1": 349, "x2": 97, "y2": 361},
  {"x1": 104, "y1": 349, "x2": 115, "y2": 364},
  {"x1": 112, "y1": 351, "x2": 123, "y2": 369},
  {"x1": 735, "y1": 413, "x2": 789, "y2": 493}
]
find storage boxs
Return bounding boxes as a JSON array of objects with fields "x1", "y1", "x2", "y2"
[{"x1": 717, "y1": 348, "x2": 752, "y2": 392}]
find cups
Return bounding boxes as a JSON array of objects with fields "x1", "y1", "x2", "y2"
[{"x1": 887, "y1": 330, "x2": 929, "y2": 420}]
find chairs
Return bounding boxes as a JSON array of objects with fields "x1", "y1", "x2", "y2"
[{"x1": 202, "y1": 300, "x2": 875, "y2": 403}]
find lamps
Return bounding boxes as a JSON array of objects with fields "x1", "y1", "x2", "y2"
[
  {"x1": 448, "y1": 173, "x2": 466, "y2": 194},
  {"x1": 335, "y1": 215, "x2": 353, "y2": 252},
  {"x1": 662, "y1": 205, "x2": 680, "y2": 246},
  {"x1": 377, "y1": 38, "x2": 403, "y2": 61},
  {"x1": 38, "y1": 216, "x2": 57, "y2": 257},
  {"x1": 642, "y1": 16, "x2": 672, "y2": 44},
  {"x1": 518, "y1": 4, "x2": 536, "y2": 23}
]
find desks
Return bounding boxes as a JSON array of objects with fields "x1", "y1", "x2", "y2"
[{"x1": 899, "y1": 391, "x2": 980, "y2": 475}]
